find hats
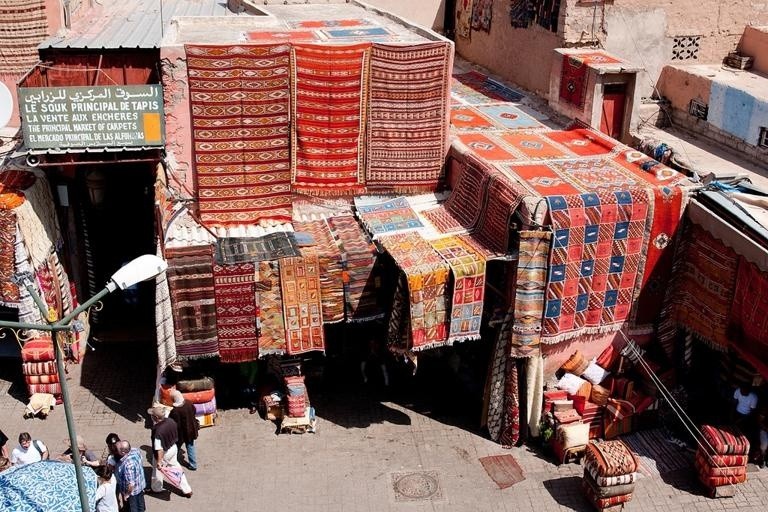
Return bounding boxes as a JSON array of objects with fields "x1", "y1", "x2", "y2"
[
  {"x1": 106, "y1": 434, "x2": 120, "y2": 444},
  {"x1": 148, "y1": 407, "x2": 165, "y2": 418}
]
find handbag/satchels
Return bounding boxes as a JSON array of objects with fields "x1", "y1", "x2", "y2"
[{"x1": 193, "y1": 417, "x2": 200, "y2": 439}]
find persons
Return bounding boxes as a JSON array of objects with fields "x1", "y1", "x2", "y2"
[
  {"x1": 156, "y1": 377, "x2": 177, "y2": 406},
  {"x1": 731, "y1": 382, "x2": 758, "y2": 418},
  {"x1": 167, "y1": 389, "x2": 200, "y2": 471},
  {"x1": 758, "y1": 402, "x2": 767, "y2": 468},
  {"x1": 0, "y1": 428, "x2": 148, "y2": 511},
  {"x1": 144, "y1": 406, "x2": 193, "y2": 499}
]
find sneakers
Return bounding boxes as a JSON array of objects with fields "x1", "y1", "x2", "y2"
[
  {"x1": 186, "y1": 492, "x2": 193, "y2": 498},
  {"x1": 145, "y1": 488, "x2": 160, "y2": 495},
  {"x1": 187, "y1": 463, "x2": 197, "y2": 470}
]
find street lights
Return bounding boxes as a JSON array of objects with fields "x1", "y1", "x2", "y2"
[{"x1": 0, "y1": 254, "x2": 168, "y2": 512}]
